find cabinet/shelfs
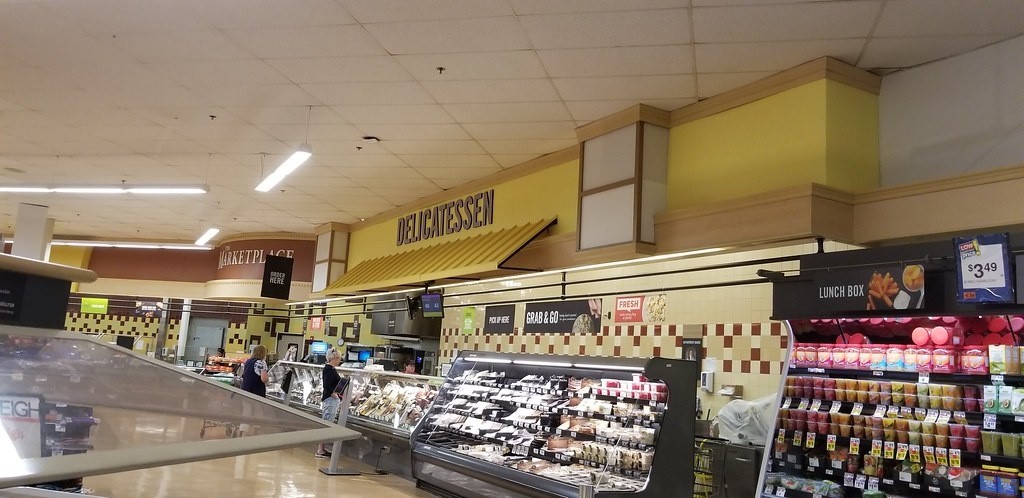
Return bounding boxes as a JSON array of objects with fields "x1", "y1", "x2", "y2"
[
  {"x1": 413, "y1": 349, "x2": 696, "y2": 497},
  {"x1": 753, "y1": 249, "x2": 1024, "y2": 497},
  {"x1": 695, "y1": 437, "x2": 765, "y2": 498},
  {"x1": 265, "y1": 360, "x2": 445, "y2": 482}
]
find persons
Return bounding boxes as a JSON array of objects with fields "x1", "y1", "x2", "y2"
[
  {"x1": 215, "y1": 347, "x2": 226, "y2": 357},
  {"x1": 314, "y1": 346, "x2": 342, "y2": 459},
  {"x1": 238, "y1": 345, "x2": 269, "y2": 438},
  {"x1": 402, "y1": 360, "x2": 421, "y2": 374}
]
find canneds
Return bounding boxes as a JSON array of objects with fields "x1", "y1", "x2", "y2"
[{"x1": 790, "y1": 316, "x2": 1024, "y2": 376}]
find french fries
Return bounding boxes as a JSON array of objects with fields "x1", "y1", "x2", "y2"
[{"x1": 866, "y1": 273, "x2": 899, "y2": 310}]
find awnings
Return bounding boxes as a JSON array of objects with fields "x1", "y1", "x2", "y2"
[{"x1": 322, "y1": 211, "x2": 565, "y2": 304}]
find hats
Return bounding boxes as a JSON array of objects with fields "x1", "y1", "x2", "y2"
[{"x1": 218, "y1": 348, "x2": 225, "y2": 354}]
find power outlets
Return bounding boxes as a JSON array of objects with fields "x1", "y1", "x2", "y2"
[{"x1": 382, "y1": 445, "x2": 391, "y2": 454}]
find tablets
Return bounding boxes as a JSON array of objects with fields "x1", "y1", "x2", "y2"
[{"x1": 333, "y1": 377, "x2": 349, "y2": 394}]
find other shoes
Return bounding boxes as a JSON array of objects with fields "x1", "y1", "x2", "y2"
[{"x1": 315, "y1": 449, "x2": 331, "y2": 458}]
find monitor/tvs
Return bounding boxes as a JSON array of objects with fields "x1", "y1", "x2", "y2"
[
  {"x1": 421, "y1": 294, "x2": 444, "y2": 319},
  {"x1": 345, "y1": 345, "x2": 374, "y2": 365},
  {"x1": 406, "y1": 295, "x2": 413, "y2": 319},
  {"x1": 312, "y1": 342, "x2": 327, "y2": 356}
]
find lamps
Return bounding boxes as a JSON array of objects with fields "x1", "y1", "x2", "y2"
[
  {"x1": 1, "y1": 183, "x2": 211, "y2": 194},
  {"x1": 3, "y1": 237, "x2": 214, "y2": 250},
  {"x1": 254, "y1": 143, "x2": 312, "y2": 192}
]
